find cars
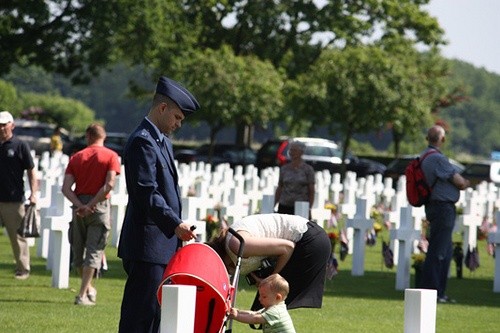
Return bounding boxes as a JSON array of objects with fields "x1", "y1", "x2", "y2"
[
  {"x1": 12, "y1": 121, "x2": 87, "y2": 158},
  {"x1": 175, "y1": 143, "x2": 257, "y2": 174},
  {"x1": 77, "y1": 132, "x2": 130, "y2": 165},
  {"x1": 255, "y1": 138, "x2": 347, "y2": 177}
]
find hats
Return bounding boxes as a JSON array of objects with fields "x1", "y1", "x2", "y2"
[
  {"x1": 0, "y1": 111, "x2": 14, "y2": 124},
  {"x1": 156, "y1": 76, "x2": 199, "y2": 117}
]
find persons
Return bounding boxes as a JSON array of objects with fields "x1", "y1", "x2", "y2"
[
  {"x1": 60, "y1": 121, "x2": 121, "y2": 305},
  {"x1": 418, "y1": 124, "x2": 471, "y2": 303},
  {"x1": 203, "y1": 212, "x2": 331, "y2": 312},
  {"x1": 227, "y1": 273, "x2": 297, "y2": 333},
  {"x1": 117, "y1": 75, "x2": 201, "y2": 332},
  {"x1": 42, "y1": 134, "x2": 71, "y2": 179},
  {"x1": 0, "y1": 110, "x2": 38, "y2": 279},
  {"x1": 56, "y1": 126, "x2": 90, "y2": 159},
  {"x1": 273, "y1": 140, "x2": 315, "y2": 222}
]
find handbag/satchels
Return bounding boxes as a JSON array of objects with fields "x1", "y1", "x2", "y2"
[{"x1": 16, "y1": 203, "x2": 41, "y2": 238}]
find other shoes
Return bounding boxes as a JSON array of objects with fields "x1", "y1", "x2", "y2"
[
  {"x1": 74, "y1": 293, "x2": 95, "y2": 306},
  {"x1": 15, "y1": 270, "x2": 30, "y2": 281},
  {"x1": 86, "y1": 286, "x2": 97, "y2": 302},
  {"x1": 439, "y1": 296, "x2": 455, "y2": 302}
]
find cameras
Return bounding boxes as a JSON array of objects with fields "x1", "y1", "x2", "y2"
[{"x1": 246, "y1": 256, "x2": 276, "y2": 286}]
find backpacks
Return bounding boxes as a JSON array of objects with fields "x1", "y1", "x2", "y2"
[{"x1": 405, "y1": 149, "x2": 442, "y2": 208}]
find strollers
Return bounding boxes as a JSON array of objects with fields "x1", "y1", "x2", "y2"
[{"x1": 156, "y1": 226, "x2": 245, "y2": 333}]
